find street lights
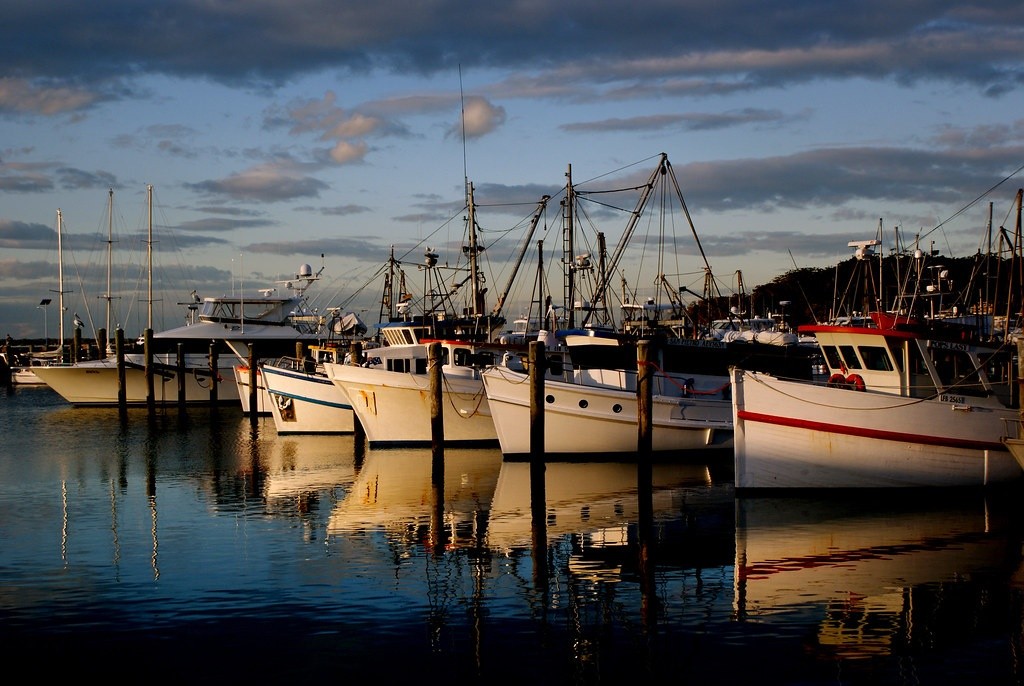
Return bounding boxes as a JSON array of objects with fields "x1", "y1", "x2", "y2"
[{"x1": 36, "y1": 299, "x2": 53, "y2": 352}]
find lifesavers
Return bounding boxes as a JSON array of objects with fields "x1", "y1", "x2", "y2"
[
  {"x1": 829, "y1": 374, "x2": 847, "y2": 389},
  {"x1": 846, "y1": 374, "x2": 864, "y2": 391}
]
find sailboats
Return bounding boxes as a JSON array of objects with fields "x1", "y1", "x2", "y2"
[{"x1": 1, "y1": 63, "x2": 1024, "y2": 496}]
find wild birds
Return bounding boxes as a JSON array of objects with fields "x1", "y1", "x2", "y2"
[{"x1": 190, "y1": 289, "x2": 200, "y2": 304}]
